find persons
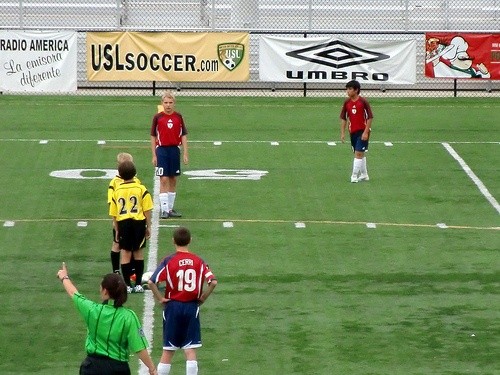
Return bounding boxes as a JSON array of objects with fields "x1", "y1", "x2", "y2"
[
  {"x1": 109, "y1": 161, "x2": 154, "y2": 293},
  {"x1": 107, "y1": 153, "x2": 141, "y2": 280},
  {"x1": 340, "y1": 80, "x2": 373, "y2": 182},
  {"x1": 150, "y1": 93, "x2": 188, "y2": 219},
  {"x1": 148, "y1": 227, "x2": 217, "y2": 375},
  {"x1": 57, "y1": 262, "x2": 158, "y2": 375}
]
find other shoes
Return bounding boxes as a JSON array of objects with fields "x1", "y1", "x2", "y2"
[
  {"x1": 134, "y1": 284, "x2": 144, "y2": 292},
  {"x1": 358, "y1": 174, "x2": 370, "y2": 181},
  {"x1": 161, "y1": 211, "x2": 169, "y2": 219},
  {"x1": 350, "y1": 173, "x2": 358, "y2": 182},
  {"x1": 169, "y1": 209, "x2": 182, "y2": 217},
  {"x1": 126, "y1": 286, "x2": 133, "y2": 293}
]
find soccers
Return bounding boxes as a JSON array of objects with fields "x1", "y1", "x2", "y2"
[{"x1": 140, "y1": 272, "x2": 158, "y2": 290}]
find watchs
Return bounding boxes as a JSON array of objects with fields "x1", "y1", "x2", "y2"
[{"x1": 61, "y1": 275, "x2": 68, "y2": 282}]
